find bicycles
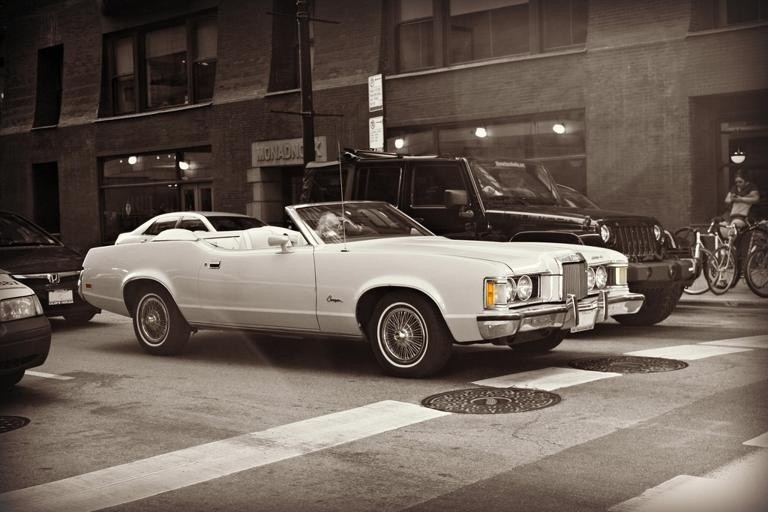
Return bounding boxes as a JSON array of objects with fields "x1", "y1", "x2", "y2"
[{"x1": 667, "y1": 217, "x2": 767, "y2": 300}]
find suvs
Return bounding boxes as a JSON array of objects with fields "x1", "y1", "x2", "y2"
[{"x1": 300, "y1": 146, "x2": 696, "y2": 330}]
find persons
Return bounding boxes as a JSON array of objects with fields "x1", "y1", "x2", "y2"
[{"x1": 723, "y1": 166, "x2": 760, "y2": 248}]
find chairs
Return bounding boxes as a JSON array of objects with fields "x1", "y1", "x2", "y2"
[{"x1": 197, "y1": 224, "x2": 303, "y2": 251}]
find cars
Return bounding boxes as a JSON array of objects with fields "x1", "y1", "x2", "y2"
[
  {"x1": 72, "y1": 197, "x2": 650, "y2": 378},
  {"x1": 0, "y1": 205, "x2": 102, "y2": 394}
]
